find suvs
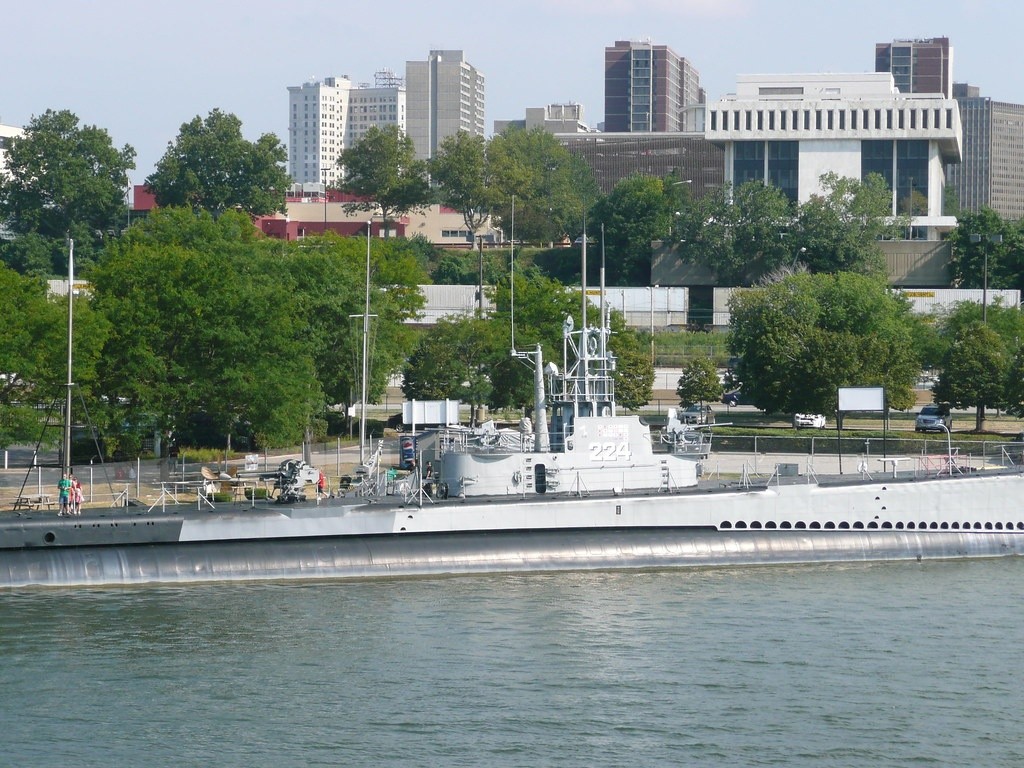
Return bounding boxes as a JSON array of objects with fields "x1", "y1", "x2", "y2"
[{"x1": 915, "y1": 404, "x2": 952, "y2": 434}]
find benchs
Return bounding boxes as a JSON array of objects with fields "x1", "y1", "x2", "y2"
[{"x1": 9, "y1": 493, "x2": 55, "y2": 511}]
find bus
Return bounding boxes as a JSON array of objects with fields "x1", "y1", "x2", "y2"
[{"x1": 64, "y1": 419, "x2": 179, "y2": 463}]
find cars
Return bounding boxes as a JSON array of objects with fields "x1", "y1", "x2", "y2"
[
  {"x1": 794, "y1": 413, "x2": 826, "y2": 430},
  {"x1": 721, "y1": 389, "x2": 756, "y2": 407},
  {"x1": 679, "y1": 404, "x2": 716, "y2": 426}
]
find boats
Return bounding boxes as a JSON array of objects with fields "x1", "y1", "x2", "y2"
[{"x1": 2, "y1": 203, "x2": 1024, "y2": 587}]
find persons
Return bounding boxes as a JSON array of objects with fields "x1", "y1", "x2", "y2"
[
  {"x1": 57, "y1": 473, "x2": 85, "y2": 516},
  {"x1": 316, "y1": 469, "x2": 330, "y2": 501},
  {"x1": 425, "y1": 460, "x2": 434, "y2": 497},
  {"x1": 707, "y1": 405, "x2": 712, "y2": 414}
]
